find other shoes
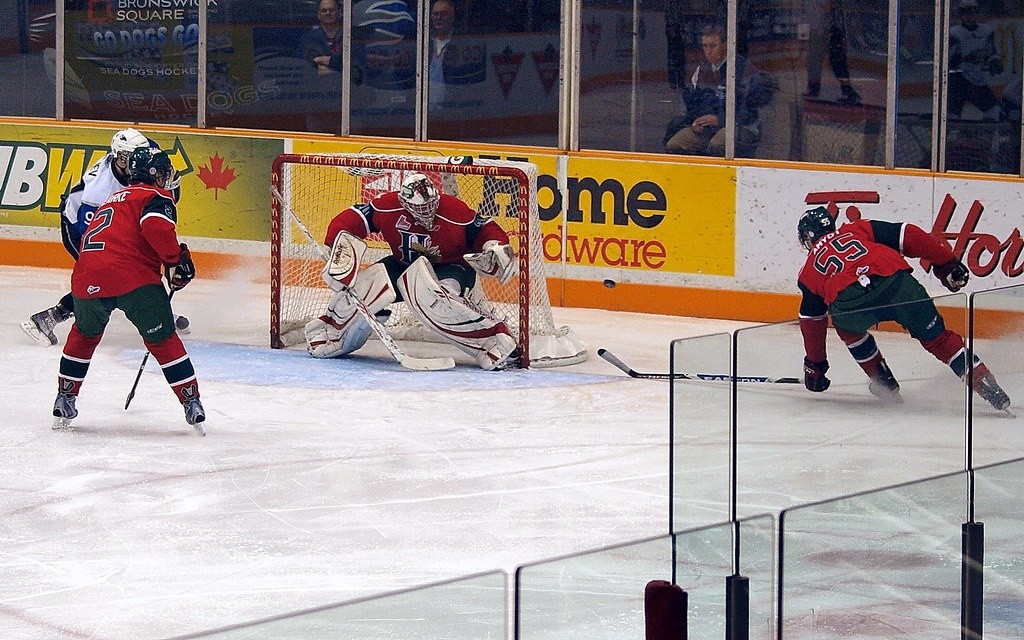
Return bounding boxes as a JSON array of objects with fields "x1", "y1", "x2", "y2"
[
  {"x1": 837, "y1": 88, "x2": 863, "y2": 103},
  {"x1": 801, "y1": 85, "x2": 820, "y2": 101}
]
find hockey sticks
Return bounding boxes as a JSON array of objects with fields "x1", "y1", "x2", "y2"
[
  {"x1": 597, "y1": 349, "x2": 804, "y2": 384},
  {"x1": 125, "y1": 289, "x2": 178, "y2": 410},
  {"x1": 269, "y1": 182, "x2": 457, "y2": 373}
]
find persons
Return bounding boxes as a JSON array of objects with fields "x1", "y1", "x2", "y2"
[
  {"x1": 30, "y1": 127, "x2": 189, "y2": 344},
  {"x1": 395, "y1": 0, "x2": 473, "y2": 140},
  {"x1": 665, "y1": 19, "x2": 773, "y2": 156},
  {"x1": 53, "y1": 147, "x2": 206, "y2": 426},
  {"x1": 665, "y1": 6, "x2": 687, "y2": 92},
  {"x1": 796, "y1": 206, "x2": 1012, "y2": 413},
  {"x1": 303, "y1": 174, "x2": 522, "y2": 372},
  {"x1": 295, "y1": 0, "x2": 367, "y2": 134},
  {"x1": 947, "y1": 1, "x2": 1023, "y2": 173},
  {"x1": 800, "y1": 0, "x2": 863, "y2": 104}
]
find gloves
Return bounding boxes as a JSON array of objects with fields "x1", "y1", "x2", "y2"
[
  {"x1": 933, "y1": 257, "x2": 971, "y2": 292},
  {"x1": 164, "y1": 243, "x2": 197, "y2": 292},
  {"x1": 802, "y1": 356, "x2": 831, "y2": 393}
]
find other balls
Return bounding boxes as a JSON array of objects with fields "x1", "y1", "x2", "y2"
[{"x1": 604, "y1": 279, "x2": 616, "y2": 288}]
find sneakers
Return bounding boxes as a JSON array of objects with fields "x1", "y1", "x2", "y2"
[
  {"x1": 21, "y1": 307, "x2": 61, "y2": 348},
  {"x1": 181, "y1": 385, "x2": 206, "y2": 436},
  {"x1": 977, "y1": 371, "x2": 1019, "y2": 418},
  {"x1": 173, "y1": 314, "x2": 191, "y2": 336},
  {"x1": 868, "y1": 358, "x2": 905, "y2": 406},
  {"x1": 51, "y1": 392, "x2": 79, "y2": 432}
]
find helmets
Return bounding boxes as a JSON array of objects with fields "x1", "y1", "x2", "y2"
[
  {"x1": 111, "y1": 128, "x2": 150, "y2": 158},
  {"x1": 398, "y1": 174, "x2": 441, "y2": 205},
  {"x1": 128, "y1": 145, "x2": 173, "y2": 181},
  {"x1": 797, "y1": 206, "x2": 837, "y2": 251}
]
